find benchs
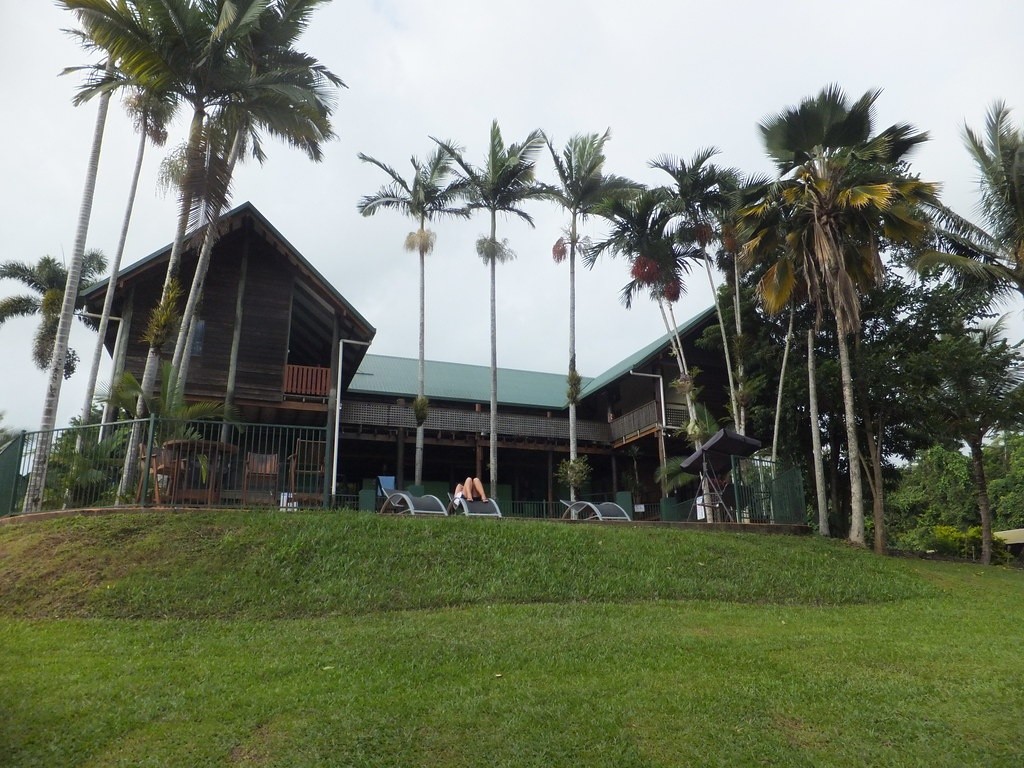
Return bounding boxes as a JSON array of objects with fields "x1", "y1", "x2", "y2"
[{"x1": 699, "y1": 483, "x2": 753, "y2": 509}]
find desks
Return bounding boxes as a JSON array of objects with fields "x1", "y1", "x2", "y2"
[{"x1": 162, "y1": 440, "x2": 239, "y2": 504}]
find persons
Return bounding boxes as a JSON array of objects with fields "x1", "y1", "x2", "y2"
[{"x1": 454, "y1": 477, "x2": 489, "y2": 506}]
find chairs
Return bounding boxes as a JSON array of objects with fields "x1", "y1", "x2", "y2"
[
  {"x1": 242, "y1": 451, "x2": 278, "y2": 507},
  {"x1": 446, "y1": 492, "x2": 503, "y2": 518},
  {"x1": 559, "y1": 499, "x2": 632, "y2": 522},
  {"x1": 136, "y1": 443, "x2": 185, "y2": 505},
  {"x1": 377, "y1": 487, "x2": 449, "y2": 517},
  {"x1": 285, "y1": 439, "x2": 328, "y2": 512}
]
